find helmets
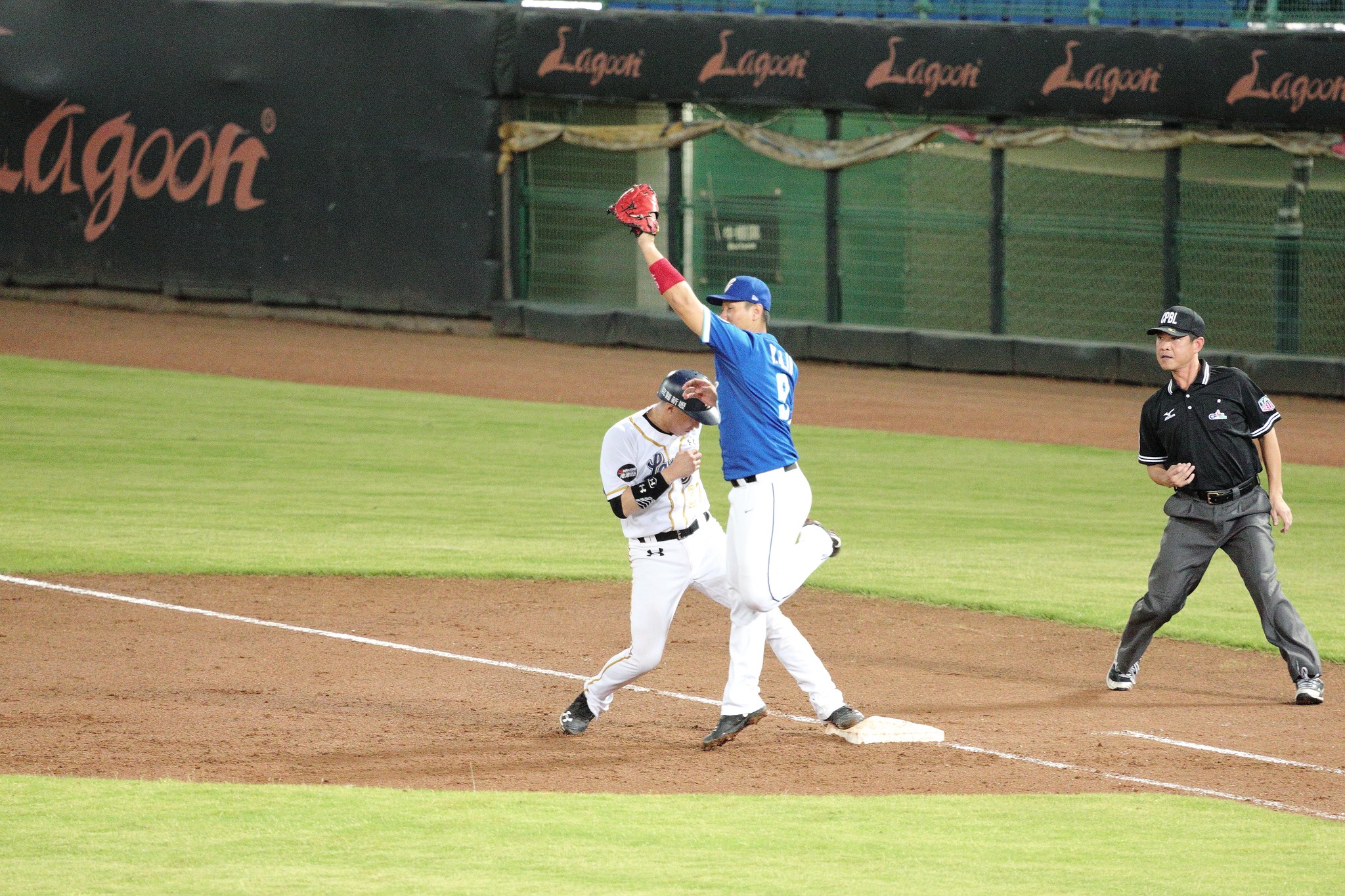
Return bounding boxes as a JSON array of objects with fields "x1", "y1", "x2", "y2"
[{"x1": 657, "y1": 370, "x2": 721, "y2": 425}]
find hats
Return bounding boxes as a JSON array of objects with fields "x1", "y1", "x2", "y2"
[
  {"x1": 1146, "y1": 306, "x2": 1205, "y2": 339},
  {"x1": 706, "y1": 275, "x2": 771, "y2": 311}
]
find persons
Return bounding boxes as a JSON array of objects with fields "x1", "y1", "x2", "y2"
[
  {"x1": 559, "y1": 369, "x2": 864, "y2": 736},
  {"x1": 1104, "y1": 306, "x2": 1325, "y2": 705},
  {"x1": 606, "y1": 183, "x2": 839, "y2": 751}
]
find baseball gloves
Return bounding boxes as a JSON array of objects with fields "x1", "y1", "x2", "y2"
[{"x1": 605, "y1": 182, "x2": 660, "y2": 236}]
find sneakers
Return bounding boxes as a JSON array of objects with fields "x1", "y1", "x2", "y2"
[
  {"x1": 824, "y1": 705, "x2": 864, "y2": 730},
  {"x1": 559, "y1": 694, "x2": 594, "y2": 735},
  {"x1": 703, "y1": 704, "x2": 767, "y2": 747},
  {"x1": 1105, "y1": 641, "x2": 1142, "y2": 690},
  {"x1": 1293, "y1": 660, "x2": 1325, "y2": 705},
  {"x1": 803, "y1": 518, "x2": 841, "y2": 557}
]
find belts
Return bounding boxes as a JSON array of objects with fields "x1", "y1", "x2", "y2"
[
  {"x1": 638, "y1": 511, "x2": 709, "y2": 543},
  {"x1": 1174, "y1": 477, "x2": 1259, "y2": 505},
  {"x1": 731, "y1": 462, "x2": 797, "y2": 487}
]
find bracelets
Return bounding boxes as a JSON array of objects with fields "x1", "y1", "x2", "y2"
[{"x1": 649, "y1": 258, "x2": 686, "y2": 295}]
